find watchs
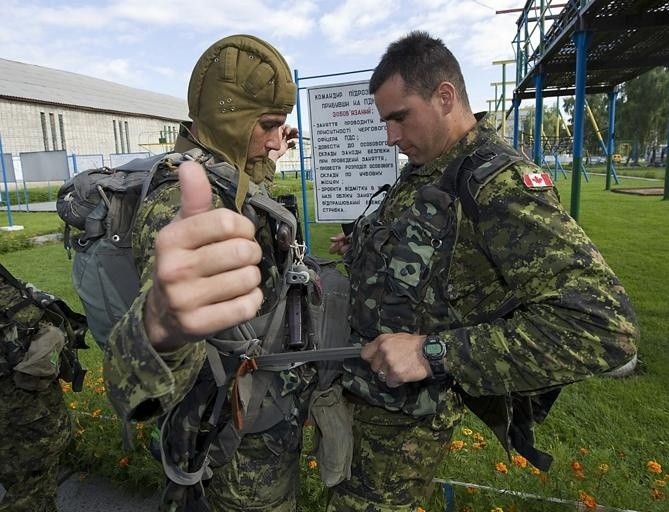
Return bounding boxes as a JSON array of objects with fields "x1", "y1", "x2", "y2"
[{"x1": 422, "y1": 331, "x2": 449, "y2": 381}]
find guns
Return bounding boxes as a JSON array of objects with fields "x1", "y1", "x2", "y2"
[{"x1": 275, "y1": 194, "x2": 307, "y2": 350}]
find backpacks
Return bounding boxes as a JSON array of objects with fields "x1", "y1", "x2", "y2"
[{"x1": 56, "y1": 146, "x2": 172, "y2": 426}]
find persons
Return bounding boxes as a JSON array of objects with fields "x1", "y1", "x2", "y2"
[
  {"x1": 0, "y1": 264, "x2": 90, "y2": 512},
  {"x1": 322, "y1": 32, "x2": 642, "y2": 512},
  {"x1": 101, "y1": 32, "x2": 323, "y2": 510}
]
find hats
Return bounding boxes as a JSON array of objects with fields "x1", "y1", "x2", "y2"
[{"x1": 187, "y1": 32, "x2": 297, "y2": 170}]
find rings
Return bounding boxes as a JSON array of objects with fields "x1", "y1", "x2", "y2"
[{"x1": 375, "y1": 366, "x2": 387, "y2": 380}]
